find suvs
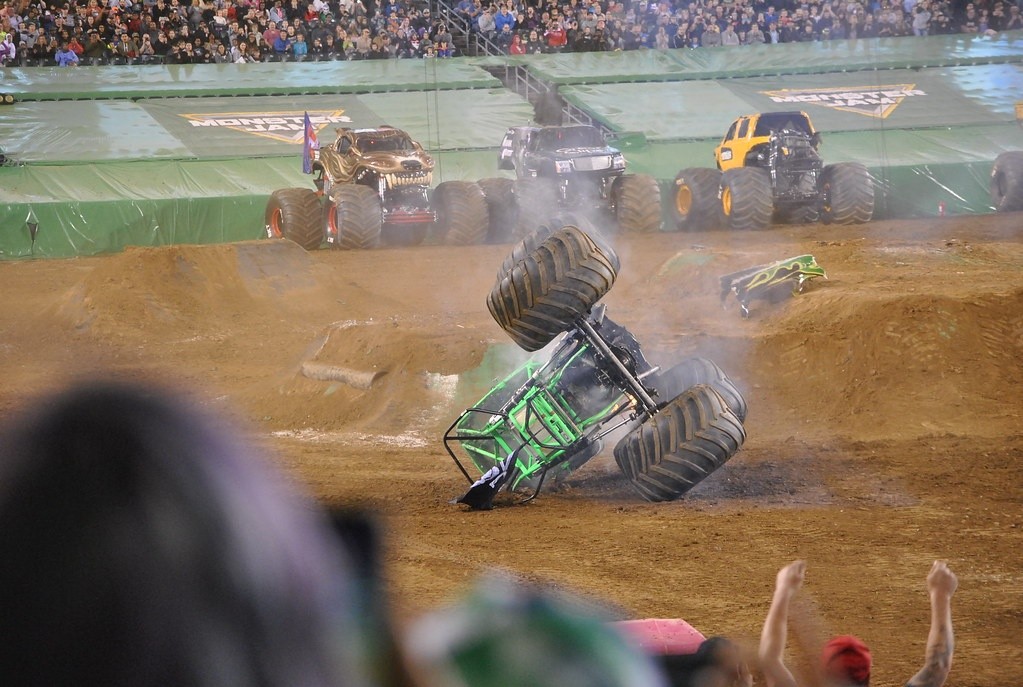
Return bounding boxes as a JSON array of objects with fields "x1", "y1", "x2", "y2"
[
  {"x1": 670, "y1": 109, "x2": 877, "y2": 242},
  {"x1": 497, "y1": 122, "x2": 662, "y2": 244},
  {"x1": 262, "y1": 124, "x2": 490, "y2": 248},
  {"x1": 988, "y1": 102, "x2": 1022, "y2": 215},
  {"x1": 434, "y1": 213, "x2": 746, "y2": 512}
]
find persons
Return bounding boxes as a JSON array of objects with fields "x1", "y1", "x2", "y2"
[
  {"x1": 758, "y1": 560, "x2": 958, "y2": 687},
  {"x1": 0, "y1": 0, "x2": 456, "y2": 67},
  {"x1": 458, "y1": 0, "x2": 1023, "y2": 54},
  {"x1": 0, "y1": 374, "x2": 365, "y2": 687},
  {"x1": 692, "y1": 637, "x2": 755, "y2": 687}
]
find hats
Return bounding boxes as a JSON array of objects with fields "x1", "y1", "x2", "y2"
[
  {"x1": 132, "y1": 32, "x2": 139, "y2": 37},
  {"x1": 821, "y1": 635, "x2": 871, "y2": 685},
  {"x1": 80, "y1": 3, "x2": 86, "y2": 8}
]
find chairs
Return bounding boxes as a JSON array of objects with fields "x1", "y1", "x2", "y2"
[{"x1": 16, "y1": 9, "x2": 465, "y2": 67}]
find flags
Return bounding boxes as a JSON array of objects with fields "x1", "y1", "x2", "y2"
[
  {"x1": 447, "y1": 445, "x2": 518, "y2": 510},
  {"x1": 303, "y1": 112, "x2": 320, "y2": 174}
]
